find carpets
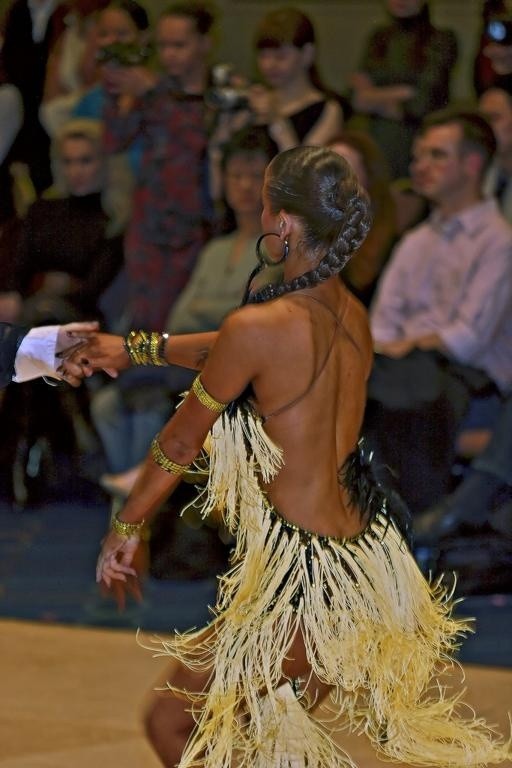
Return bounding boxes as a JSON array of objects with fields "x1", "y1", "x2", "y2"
[{"x1": 0, "y1": 500, "x2": 512, "y2": 670}]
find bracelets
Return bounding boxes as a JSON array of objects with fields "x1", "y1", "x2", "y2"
[
  {"x1": 112, "y1": 510, "x2": 146, "y2": 536},
  {"x1": 124, "y1": 328, "x2": 169, "y2": 368}
]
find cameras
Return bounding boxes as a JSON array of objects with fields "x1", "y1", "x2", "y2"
[{"x1": 206, "y1": 63, "x2": 247, "y2": 111}]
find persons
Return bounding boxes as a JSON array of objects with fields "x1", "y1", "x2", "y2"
[
  {"x1": 3, "y1": 1, "x2": 512, "y2": 604},
  {"x1": 57, "y1": 145, "x2": 511, "y2": 766},
  {"x1": 0, "y1": 317, "x2": 119, "y2": 388}
]
